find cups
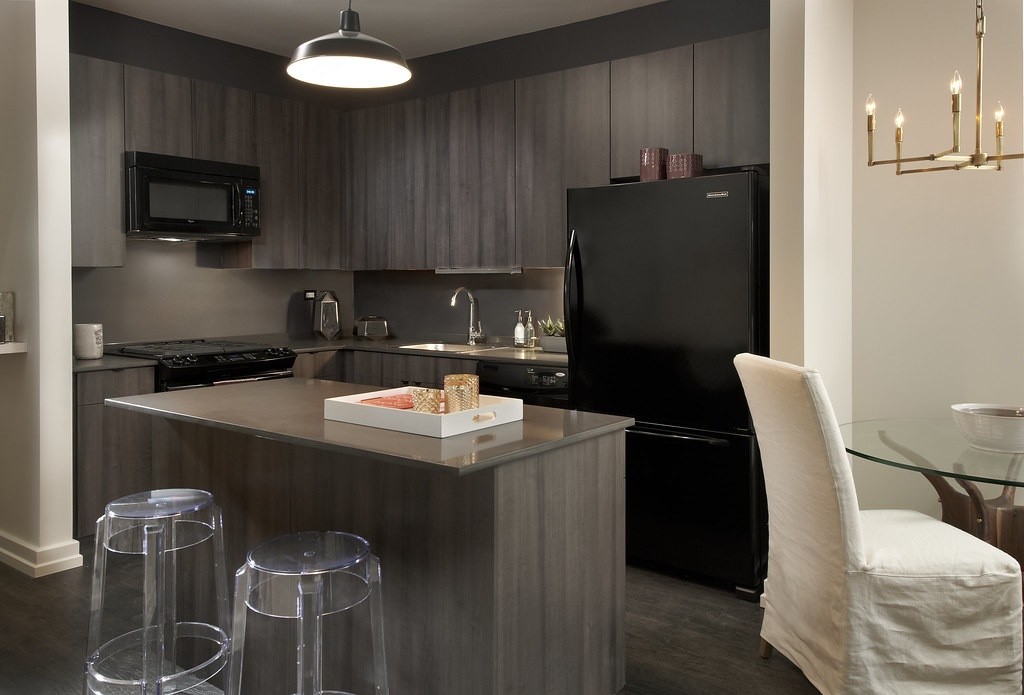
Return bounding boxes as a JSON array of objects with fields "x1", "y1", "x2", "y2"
[
  {"x1": 412, "y1": 388, "x2": 441, "y2": 415},
  {"x1": 72, "y1": 323, "x2": 104, "y2": 360},
  {"x1": 443, "y1": 373, "x2": 480, "y2": 415}
]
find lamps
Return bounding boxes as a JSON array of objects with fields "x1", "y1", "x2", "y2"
[
  {"x1": 866, "y1": 0, "x2": 1024, "y2": 175},
  {"x1": 284, "y1": 0, "x2": 413, "y2": 89}
]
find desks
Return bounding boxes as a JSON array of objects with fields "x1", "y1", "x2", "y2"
[
  {"x1": 838, "y1": 417, "x2": 1024, "y2": 565},
  {"x1": 103, "y1": 376, "x2": 637, "y2": 695}
]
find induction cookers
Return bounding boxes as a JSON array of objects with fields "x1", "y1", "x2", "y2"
[{"x1": 102, "y1": 338, "x2": 299, "y2": 394}]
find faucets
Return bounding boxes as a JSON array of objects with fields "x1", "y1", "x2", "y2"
[{"x1": 450, "y1": 287, "x2": 487, "y2": 345}]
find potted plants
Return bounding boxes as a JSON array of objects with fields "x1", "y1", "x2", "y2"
[{"x1": 537, "y1": 315, "x2": 567, "y2": 353}]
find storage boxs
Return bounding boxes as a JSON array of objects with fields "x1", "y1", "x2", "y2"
[{"x1": 324, "y1": 383, "x2": 523, "y2": 439}]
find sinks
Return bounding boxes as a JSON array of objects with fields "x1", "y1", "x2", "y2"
[{"x1": 399, "y1": 340, "x2": 502, "y2": 353}]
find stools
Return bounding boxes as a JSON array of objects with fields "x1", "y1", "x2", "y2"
[
  {"x1": 83, "y1": 488, "x2": 231, "y2": 695},
  {"x1": 228, "y1": 530, "x2": 388, "y2": 695}
]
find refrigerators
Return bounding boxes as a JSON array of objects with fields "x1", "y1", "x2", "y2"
[{"x1": 563, "y1": 166, "x2": 775, "y2": 602}]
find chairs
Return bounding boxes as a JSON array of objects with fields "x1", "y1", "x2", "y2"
[{"x1": 734, "y1": 353, "x2": 1022, "y2": 695}]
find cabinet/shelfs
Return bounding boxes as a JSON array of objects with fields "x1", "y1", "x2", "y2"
[
  {"x1": 72, "y1": 329, "x2": 569, "y2": 539},
  {"x1": 70, "y1": 30, "x2": 771, "y2": 267}
]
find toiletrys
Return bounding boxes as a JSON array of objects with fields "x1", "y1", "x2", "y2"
[
  {"x1": 522, "y1": 310, "x2": 535, "y2": 348},
  {"x1": 513, "y1": 309, "x2": 524, "y2": 347}
]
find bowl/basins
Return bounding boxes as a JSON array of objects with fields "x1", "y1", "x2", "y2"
[{"x1": 950, "y1": 403, "x2": 1024, "y2": 454}]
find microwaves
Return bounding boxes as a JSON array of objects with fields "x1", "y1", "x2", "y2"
[{"x1": 122, "y1": 150, "x2": 263, "y2": 245}]
